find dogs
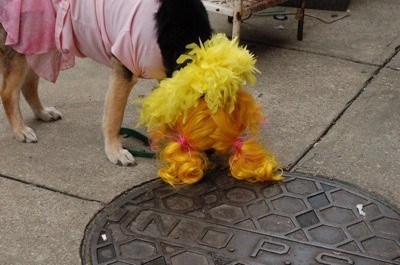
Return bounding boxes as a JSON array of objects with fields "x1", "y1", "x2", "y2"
[{"x1": 0, "y1": 0, "x2": 247, "y2": 167}]
